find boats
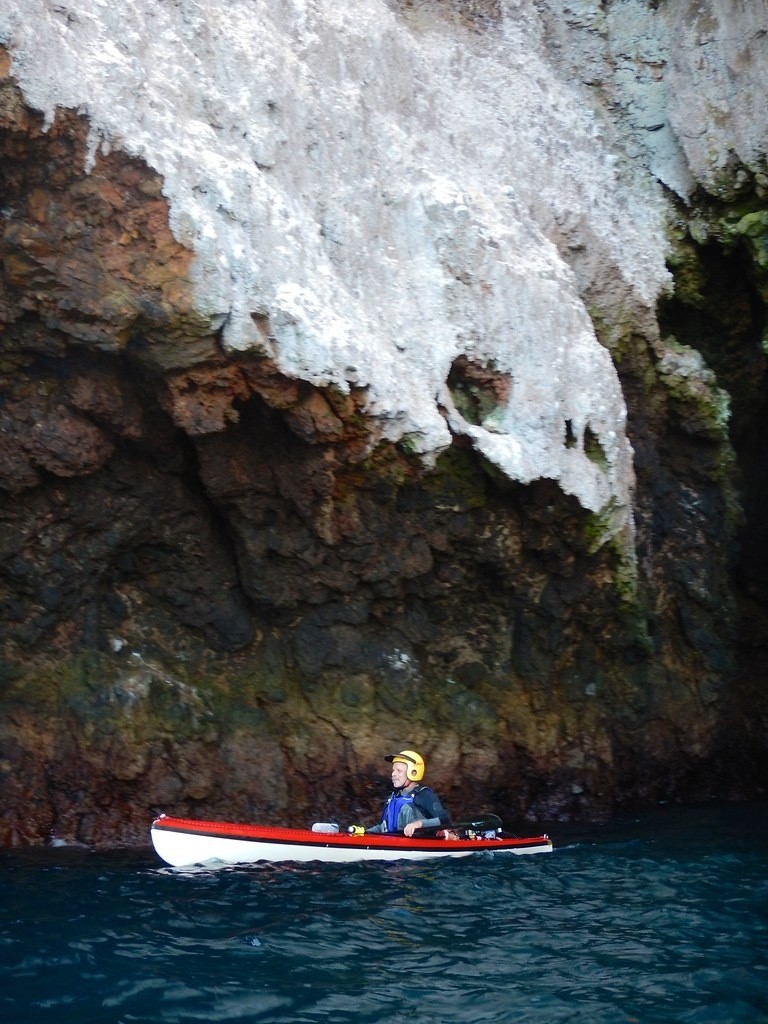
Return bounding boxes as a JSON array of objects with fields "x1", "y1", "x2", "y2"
[{"x1": 151, "y1": 813, "x2": 553, "y2": 868}]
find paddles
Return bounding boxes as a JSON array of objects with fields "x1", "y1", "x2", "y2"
[{"x1": 309, "y1": 812, "x2": 505, "y2": 836}]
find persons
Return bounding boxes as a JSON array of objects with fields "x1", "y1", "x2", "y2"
[{"x1": 365, "y1": 751, "x2": 450, "y2": 839}]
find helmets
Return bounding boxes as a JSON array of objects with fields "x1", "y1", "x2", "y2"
[{"x1": 384, "y1": 750, "x2": 425, "y2": 781}]
